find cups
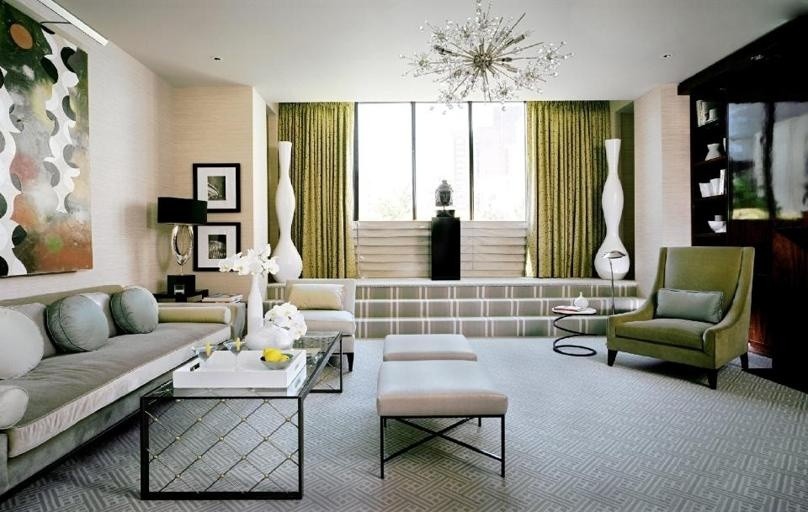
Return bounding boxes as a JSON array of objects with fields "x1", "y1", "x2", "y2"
[
  {"x1": 174, "y1": 283, "x2": 184, "y2": 295},
  {"x1": 569, "y1": 298, "x2": 576, "y2": 306}
]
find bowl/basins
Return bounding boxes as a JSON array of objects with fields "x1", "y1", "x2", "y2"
[{"x1": 259, "y1": 353, "x2": 294, "y2": 370}]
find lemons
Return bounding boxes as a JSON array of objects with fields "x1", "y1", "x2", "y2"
[
  {"x1": 235, "y1": 338, "x2": 241, "y2": 351},
  {"x1": 205, "y1": 341, "x2": 209, "y2": 356},
  {"x1": 261, "y1": 348, "x2": 289, "y2": 362}
]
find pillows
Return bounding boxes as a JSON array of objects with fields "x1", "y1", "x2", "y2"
[
  {"x1": 652, "y1": 284, "x2": 727, "y2": 325},
  {"x1": 110, "y1": 285, "x2": 161, "y2": 338},
  {"x1": 287, "y1": 283, "x2": 349, "y2": 311},
  {"x1": 46, "y1": 294, "x2": 112, "y2": 353},
  {"x1": 1, "y1": 306, "x2": 45, "y2": 383}
]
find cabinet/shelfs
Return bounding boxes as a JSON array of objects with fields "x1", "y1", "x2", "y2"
[
  {"x1": 427, "y1": 215, "x2": 461, "y2": 281},
  {"x1": 673, "y1": 15, "x2": 807, "y2": 237},
  {"x1": 691, "y1": 237, "x2": 806, "y2": 391}
]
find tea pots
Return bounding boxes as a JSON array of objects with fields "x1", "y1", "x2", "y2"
[{"x1": 574, "y1": 292, "x2": 591, "y2": 310}]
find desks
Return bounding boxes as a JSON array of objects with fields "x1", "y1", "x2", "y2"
[
  {"x1": 153, "y1": 290, "x2": 209, "y2": 304},
  {"x1": 547, "y1": 306, "x2": 598, "y2": 357}
]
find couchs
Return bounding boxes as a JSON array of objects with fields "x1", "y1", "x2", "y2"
[
  {"x1": 382, "y1": 334, "x2": 478, "y2": 360},
  {"x1": 375, "y1": 360, "x2": 509, "y2": 480},
  {"x1": 282, "y1": 278, "x2": 357, "y2": 373},
  {"x1": 0, "y1": 282, "x2": 245, "y2": 501},
  {"x1": 604, "y1": 244, "x2": 756, "y2": 391}
]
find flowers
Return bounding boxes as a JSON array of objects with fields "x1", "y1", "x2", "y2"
[
  {"x1": 217, "y1": 243, "x2": 282, "y2": 278},
  {"x1": 263, "y1": 302, "x2": 308, "y2": 342}
]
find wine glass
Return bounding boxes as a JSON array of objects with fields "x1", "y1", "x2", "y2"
[
  {"x1": 224, "y1": 339, "x2": 247, "y2": 373},
  {"x1": 191, "y1": 343, "x2": 218, "y2": 391}
]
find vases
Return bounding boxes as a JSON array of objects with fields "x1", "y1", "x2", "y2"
[
  {"x1": 593, "y1": 137, "x2": 632, "y2": 282},
  {"x1": 245, "y1": 274, "x2": 262, "y2": 333},
  {"x1": 265, "y1": 140, "x2": 306, "y2": 286}
]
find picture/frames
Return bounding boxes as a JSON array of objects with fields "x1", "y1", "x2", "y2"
[
  {"x1": 190, "y1": 161, "x2": 241, "y2": 215},
  {"x1": 193, "y1": 222, "x2": 241, "y2": 272}
]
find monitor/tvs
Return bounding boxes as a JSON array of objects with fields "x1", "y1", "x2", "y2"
[{"x1": 722, "y1": 92, "x2": 808, "y2": 235}]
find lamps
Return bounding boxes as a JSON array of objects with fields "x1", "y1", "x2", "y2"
[
  {"x1": 397, "y1": 0, "x2": 582, "y2": 115},
  {"x1": 150, "y1": 196, "x2": 208, "y2": 297}
]
